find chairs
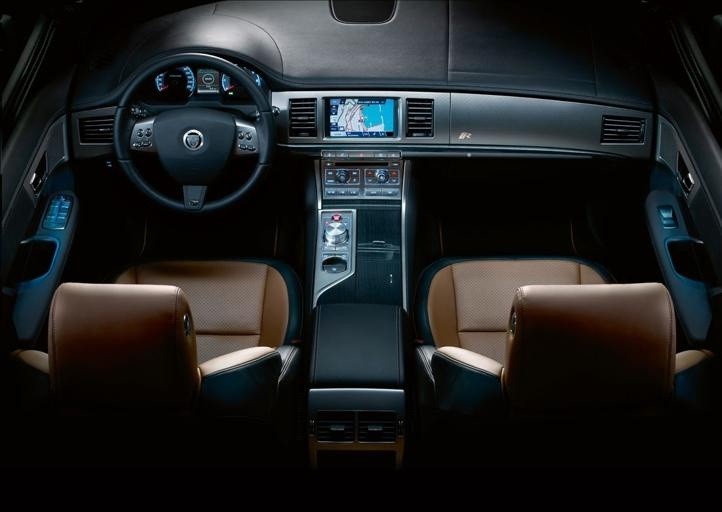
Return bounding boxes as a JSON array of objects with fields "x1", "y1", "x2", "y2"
[
  {"x1": 11, "y1": 259, "x2": 299, "y2": 414},
  {"x1": 413, "y1": 260, "x2": 712, "y2": 413}
]
